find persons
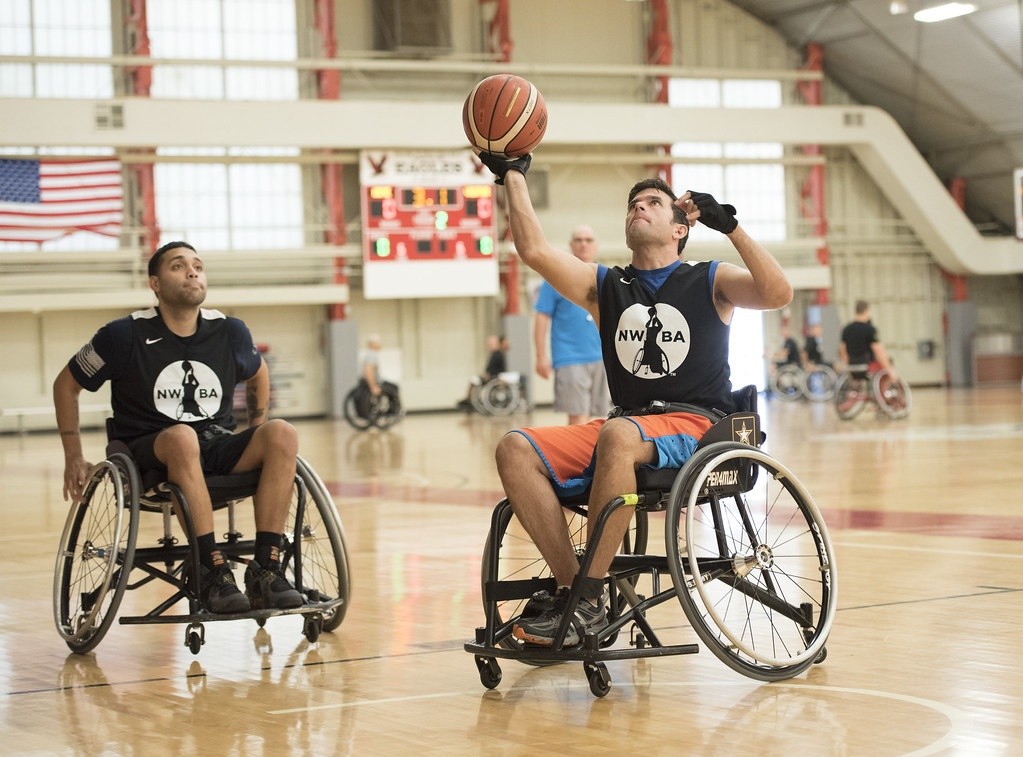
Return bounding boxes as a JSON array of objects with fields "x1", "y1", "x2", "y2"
[
  {"x1": 457, "y1": 334, "x2": 534, "y2": 414},
  {"x1": 837, "y1": 299, "x2": 898, "y2": 383},
  {"x1": 472, "y1": 144, "x2": 795, "y2": 648},
  {"x1": 765, "y1": 326, "x2": 801, "y2": 393},
  {"x1": 801, "y1": 325, "x2": 838, "y2": 389},
  {"x1": 359, "y1": 334, "x2": 401, "y2": 416},
  {"x1": 535, "y1": 225, "x2": 611, "y2": 427},
  {"x1": 53, "y1": 243, "x2": 304, "y2": 614}
]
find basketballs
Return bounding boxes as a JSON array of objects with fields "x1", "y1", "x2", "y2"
[{"x1": 462, "y1": 71, "x2": 549, "y2": 159}]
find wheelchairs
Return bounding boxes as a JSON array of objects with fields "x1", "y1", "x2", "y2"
[
  {"x1": 52, "y1": 417, "x2": 353, "y2": 655},
  {"x1": 343, "y1": 376, "x2": 406, "y2": 432},
  {"x1": 770, "y1": 361, "x2": 840, "y2": 402},
  {"x1": 463, "y1": 384, "x2": 839, "y2": 697},
  {"x1": 468, "y1": 372, "x2": 521, "y2": 418},
  {"x1": 834, "y1": 363, "x2": 914, "y2": 422}
]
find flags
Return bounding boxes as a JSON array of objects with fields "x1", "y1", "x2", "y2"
[{"x1": 0, "y1": 158, "x2": 124, "y2": 243}]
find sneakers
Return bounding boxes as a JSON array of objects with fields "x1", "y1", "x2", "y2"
[
  {"x1": 513, "y1": 588, "x2": 610, "y2": 646},
  {"x1": 188, "y1": 551, "x2": 251, "y2": 613},
  {"x1": 245, "y1": 560, "x2": 303, "y2": 608}
]
